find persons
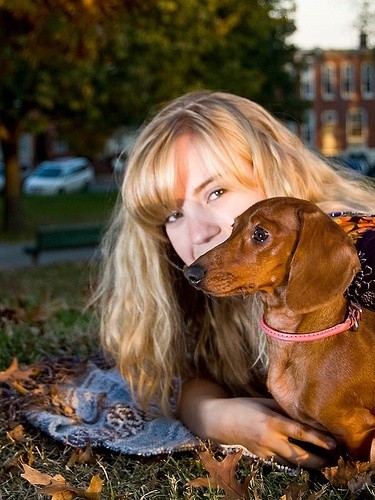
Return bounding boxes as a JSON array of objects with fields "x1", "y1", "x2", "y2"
[{"x1": 81, "y1": 90, "x2": 374, "y2": 472}]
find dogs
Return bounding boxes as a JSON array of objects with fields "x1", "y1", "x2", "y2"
[{"x1": 184, "y1": 196, "x2": 375, "y2": 466}]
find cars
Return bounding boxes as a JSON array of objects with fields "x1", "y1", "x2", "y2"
[{"x1": 22, "y1": 157, "x2": 95, "y2": 196}]
